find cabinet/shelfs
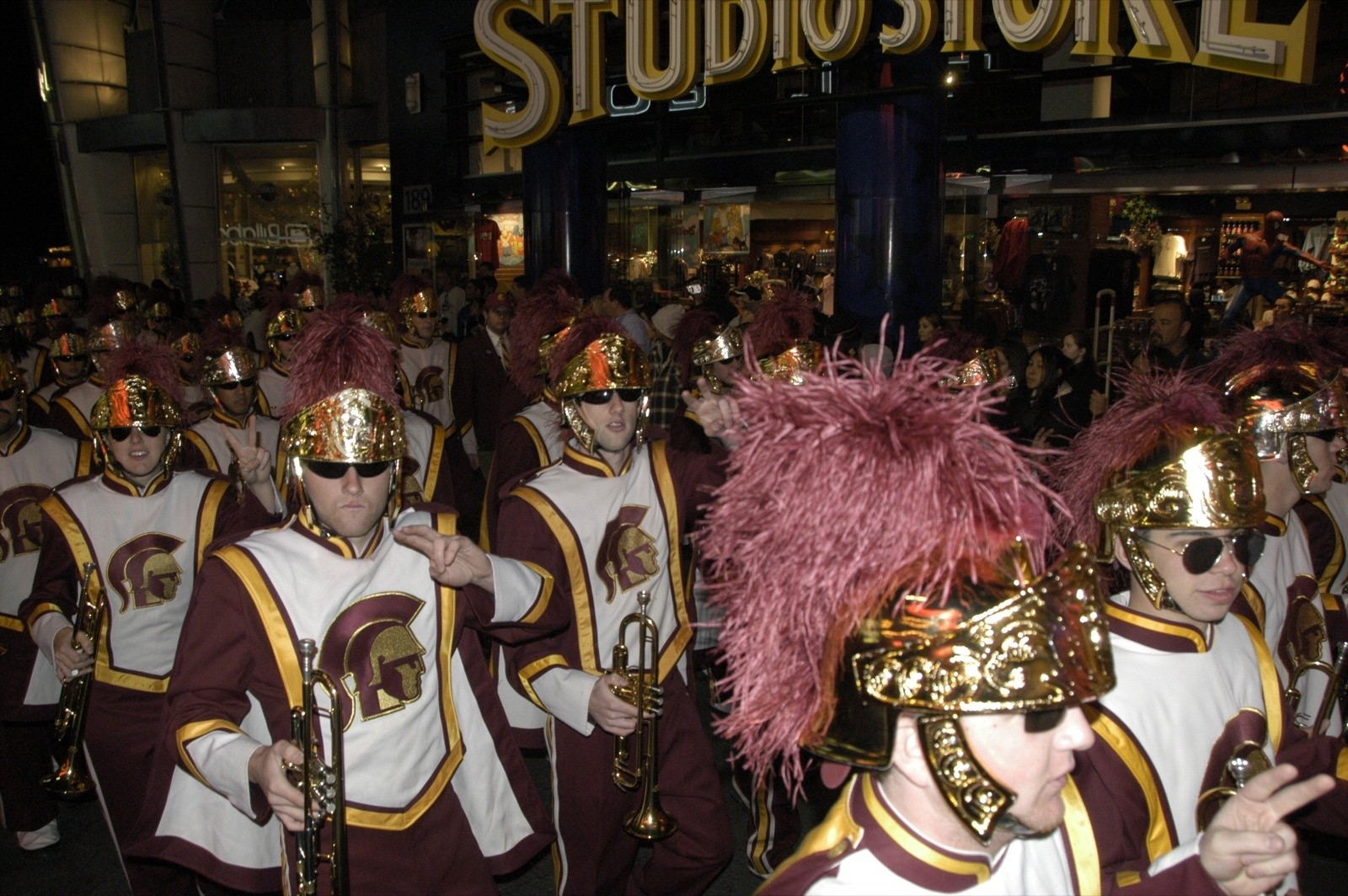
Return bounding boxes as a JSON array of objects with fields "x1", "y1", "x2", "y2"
[{"x1": 1150, "y1": 214, "x2": 1267, "y2": 304}]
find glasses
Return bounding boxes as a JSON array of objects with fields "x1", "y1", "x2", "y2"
[
  {"x1": 577, "y1": 389, "x2": 642, "y2": 404},
  {"x1": 1305, "y1": 430, "x2": 1336, "y2": 443},
  {"x1": 1134, "y1": 531, "x2": 1266, "y2": 575},
  {"x1": 110, "y1": 427, "x2": 161, "y2": 442},
  {"x1": 719, "y1": 354, "x2": 741, "y2": 365},
  {"x1": 305, "y1": 458, "x2": 390, "y2": 479},
  {"x1": 1022, "y1": 698, "x2": 1103, "y2": 733}
]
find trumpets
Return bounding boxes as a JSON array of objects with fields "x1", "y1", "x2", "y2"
[
  {"x1": 280, "y1": 638, "x2": 353, "y2": 896},
  {"x1": 41, "y1": 559, "x2": 108, "y2": 795},
  {"x1": 1284, "y1": 640, "x2": 1348, "y2": 741},
  {"x1": 609, "y1": 588, "x2": 681, "y2": 843},
  {"x1": 1196, "y1": 755, "x2": 1254, "y2": 837},
  {"x1": 228, "y1": 447, "x2": 247, "y2": 505}
]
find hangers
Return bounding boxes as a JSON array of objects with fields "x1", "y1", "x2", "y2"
[
  {"x1": 1320, "y1": 217, "x2": 1334, "y2": 229},
  {"x1": 475, "y1": 212, "x2": 489, "y2": 224}
]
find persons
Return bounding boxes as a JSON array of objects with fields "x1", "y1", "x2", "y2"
[
  {"x1": 0, "y1": 262, "x2": 1348, "y2": 896},
  {"x1": 1220, "y1": 211, "x2": 1328, "y2": 328}
]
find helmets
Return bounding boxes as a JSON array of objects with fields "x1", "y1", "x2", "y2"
[
  {"x1": 923, "y1": 328, "x2": 1012, "y2": 400},
  {"x1": 672, "y1": 313, "x2": 745, "y2": 392},
  {"x1": 553, "y1": 318, "x2": 654, "y2": 453},
  {"x1": 0, "y1": 282, "x2": 441, "y2": 435},
  {"x1": 89, "y1": 344, "x2": 187, "y2": 477},
  {"x1": 509, "y1": 289, "x2": 598, "y2": 390},
  {"x1": 282, "y1": 314, "x2": 404, "y2": 530},
  {"x1": 746, "y1": 292, "x2": 836, "y2": 392},
  {"x1": 699, "y1": 335, "x2": 1120, "y2": 849},
  {"x1": 1208, "y1": 304, "x2": 1348, "y2": 495},
  {"x1": 1048, "y1": 375, "x2": 1268, "y2": 625}
]
